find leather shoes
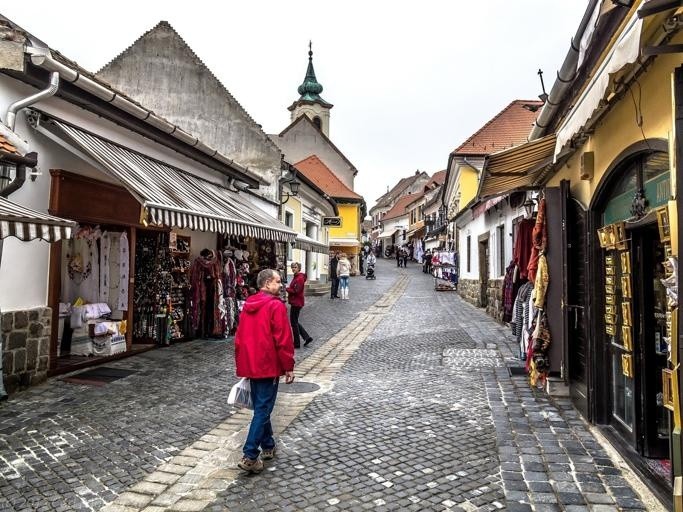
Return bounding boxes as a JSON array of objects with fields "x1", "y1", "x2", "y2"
[
  {"x1": 303, "y1": 337, "x2": 313, "y2": 346},
  {"x1": 335, "y1": 295, "x2": 340, "y2": 298},
  {"x1": 331, "y1": 295, "x2": 335, "y2": 299}
]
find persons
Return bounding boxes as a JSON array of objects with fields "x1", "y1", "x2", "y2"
[
  {"x1": 329, "y1": 250, "x2": 341, "y2": 299},
  {"x1": 365, "y1": 251, "x2": 376, "y2": 269},
  {"x1": 231, "y1": 268, "x2": 295, "y2": 477},
  {"x1": 336, "y1": 252, "x2": 352, "y2": 300},
  {"x1": 420, "y1": 250, "x2": 433, "y2": 274},
  {"x1": 395, "y1": 248, "x2": 408, "y2": 268},
  {"x1": 284, "y1": 262, "x2": 314, "y2": 349}
]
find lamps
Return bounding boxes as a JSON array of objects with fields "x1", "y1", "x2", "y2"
[
  {"x1": 281, "y1": 170, "x2": 300, "y2": 204},
  {"x1": 524, "y1": 197, "x2": 537, "y2": 219}
]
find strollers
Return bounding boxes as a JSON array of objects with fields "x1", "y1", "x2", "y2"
[{"x1": 365, "y1": 261, "x2": 376, "y2": 280}]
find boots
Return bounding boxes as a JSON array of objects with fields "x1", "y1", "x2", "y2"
[{"x1": 339, "y1": 288, "x2": 350, "y2": 300}]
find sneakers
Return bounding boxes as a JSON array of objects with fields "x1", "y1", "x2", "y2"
[
  {"x1": 260, "y1": 442, "x2": 277, "y2": 459},
  {"x1": 238, "y1": 456, "x2": 264, "y2": 474}
]
